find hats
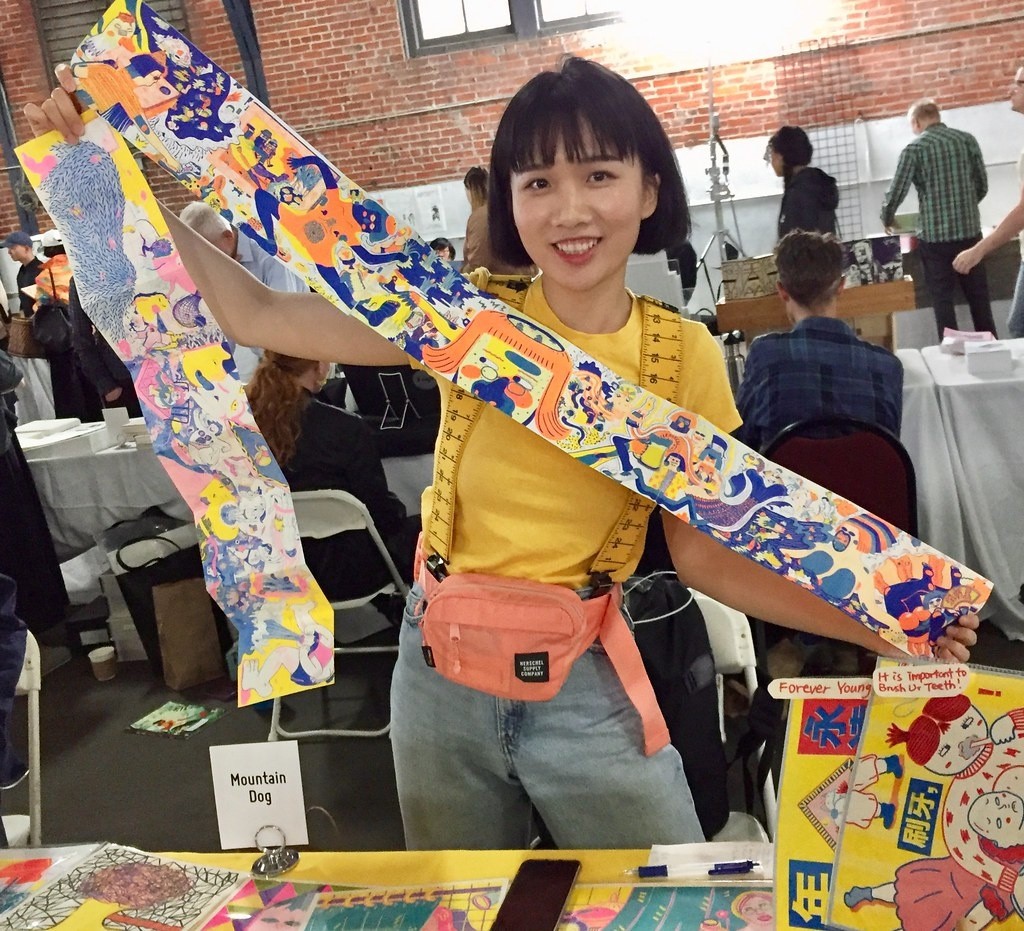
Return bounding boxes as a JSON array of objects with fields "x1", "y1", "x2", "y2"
[
  {"x1": 0, "y1": 232, "x2": 33, "y2": 248},
  {"x1": 37, "y1": 229, "x2": 63, "y2": 253}
]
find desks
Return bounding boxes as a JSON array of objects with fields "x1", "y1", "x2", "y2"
[
  {"x1": 894, "y1": 339, "x2": 1024, "y2": 642},
  {"x1": 14, "y1": 422, "x2": 198, "y2": 605}
]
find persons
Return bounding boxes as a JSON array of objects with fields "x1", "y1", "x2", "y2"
[
  {"x1": 768, "y1": 125, "x2": 840, "y2": 237},
  {"x1": 0, "y1": 346, "x2": 71, "y2": 676},
  {"x1": 25, "y1": 57, "x2": 980, "y2": 852},
  {"x1": 729, "y1": 232, "x2": 905, "y2": 455},
  {"x1": 242, "y1": 347, "x2": 422, "y2": 634},
  {"x1": 179, "y1": 199, "x2": 311, "y2": 383},
  {"x1": 33, "y1": 229, "x2": 83, "y2": 379},
  {"x1": 69, "y1": 276, "x2": 143, "y2": 423},
  {"x1": 429, "y1": 237, "x2": 454, "y2": 269},
  {"x1": 0, "y1": 231, "x2": 44, "y2": 317},
  {"x1": 878, "y1": 98, "x2": 999, "y2": 343},
  {"x1": 461, "y1": 166, "x2": 537, "y2": 277},
  {"x1": 950, "y1": 64, "x2": 1024, "y2": 340}
]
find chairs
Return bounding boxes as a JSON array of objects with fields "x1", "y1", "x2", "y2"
[
  {"x1": 0, "y1": 629, "x2": 42, "y2": 848},
  {"x1": 756, "y1": 413, "x2": 919, "y2": 675},
  {"x1": 267, "y1": 489, "x2": 411, "y2": 741},
  {"x1": 686, "y1": 587, "x2": 777, "y2": 843}
]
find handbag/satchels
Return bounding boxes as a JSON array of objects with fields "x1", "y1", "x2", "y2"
[
  {"x1": 32, "y1": 267, "x2": 74, "y2": 357},
  {"x1": 7, "y1": 313, "x2": 44, "y2": 358},
  {"x1": 413, "y1": 531, "x2": 667, "y2": 757},
  {"x1": 99, "y1": 526, "x2": 233, "y2": 690}
]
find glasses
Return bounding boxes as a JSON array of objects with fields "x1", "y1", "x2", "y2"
[{"x1": 1012, "y1": 79, "x2": 1024, "y2": 88}]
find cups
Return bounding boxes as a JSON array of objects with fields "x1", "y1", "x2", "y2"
[{"x1": 88, "y1": 646, "x2": 116, "y2": 681}]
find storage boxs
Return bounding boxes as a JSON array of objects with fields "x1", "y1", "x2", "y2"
[{"x1": 99, "y1": 569, "x2": 149, "y2": 661}]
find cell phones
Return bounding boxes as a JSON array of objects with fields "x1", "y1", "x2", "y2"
[{"x1": 490, "y1": 859, "x2": 581, "y2": 931}]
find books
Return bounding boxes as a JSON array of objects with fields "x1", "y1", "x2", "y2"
[
  {"x1": 130, "y1": 700, "x2": 232, "y2": 740},
  {"x1": 941, "y1": 326, "x2": 1012, "y2": 374}
]
